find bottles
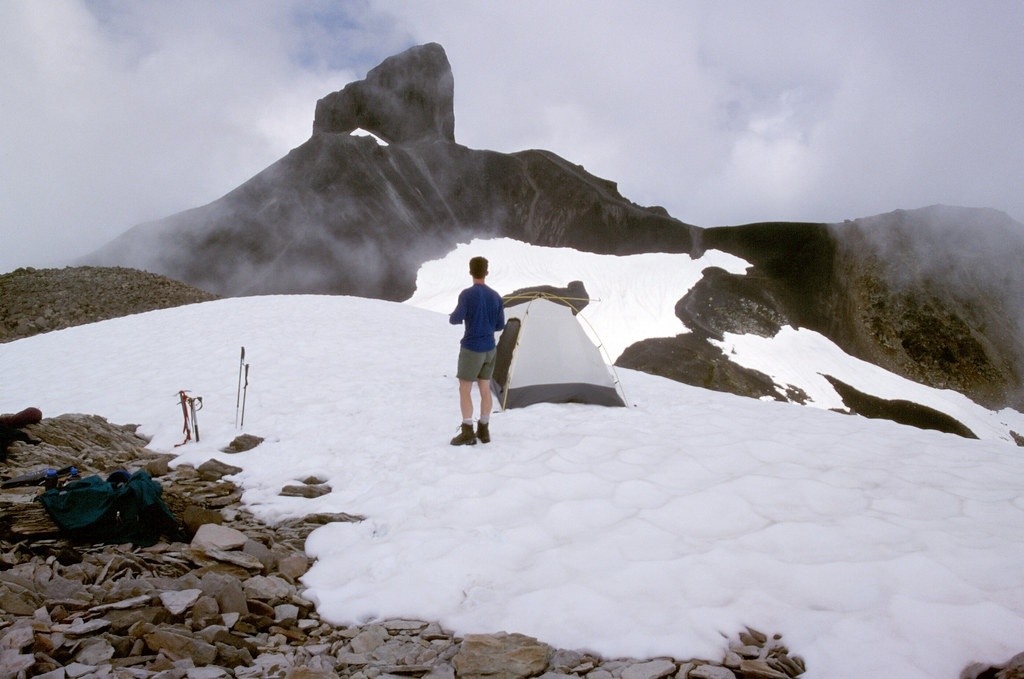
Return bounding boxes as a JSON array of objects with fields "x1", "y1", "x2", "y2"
[
  {"x1": 67, "y1": 468, "x2": 81, "y2": 480},
  {"x1": 45, "y1": 470, "x2": 58, "y2": 491}
]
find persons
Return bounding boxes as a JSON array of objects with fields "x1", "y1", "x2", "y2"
[{"x1": 449, "y1": 256, "x2": 504, "y2": 446}]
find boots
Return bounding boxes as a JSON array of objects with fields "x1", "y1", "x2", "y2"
[
  {"x1": 450, "y1": 422, "x2": 477, "y2": 446},
  {"x1": 475, "y1": 420, "x2": 490, "y2": 443}
]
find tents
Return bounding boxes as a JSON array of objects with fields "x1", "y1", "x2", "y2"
[{"x1": 489, "y1": 281, "x2": 628, "y2": 410}]
